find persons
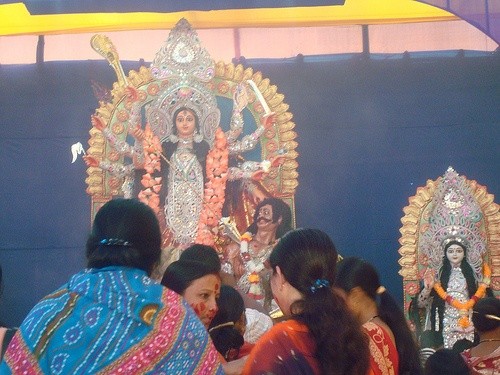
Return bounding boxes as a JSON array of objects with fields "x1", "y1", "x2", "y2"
[
  {"x1": 243, "y1": 228, "x2": 370, "y2": 375},
  {"x1": 83, "y1": 85, "x2": 285, "y2": 249},
  {"x1": 160, "y1": 244, "x2": 273, "y2": 375},
  {"x1": 0, "y1": 197, "x2": 227, "y2": 374},
  {"x1": 418, "y1": 297, "x2": 499, "y2": 375},
  {"x1": 332, "y1": 257, "x2": 423, "y2": 375},
  {"x1": 429, "y1": 240, "x2": 481, "y2": 353},
  {"x1": 224, "y1": 196, "x2": 293, "y2": 324}
]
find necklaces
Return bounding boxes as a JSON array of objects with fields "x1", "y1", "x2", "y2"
[
  {"x1": 478, "y1": 338, "x2": 499, "y2": 344},
  {"x1": 367, "y1": 315, "x2": 381, "y2": 322}
]
